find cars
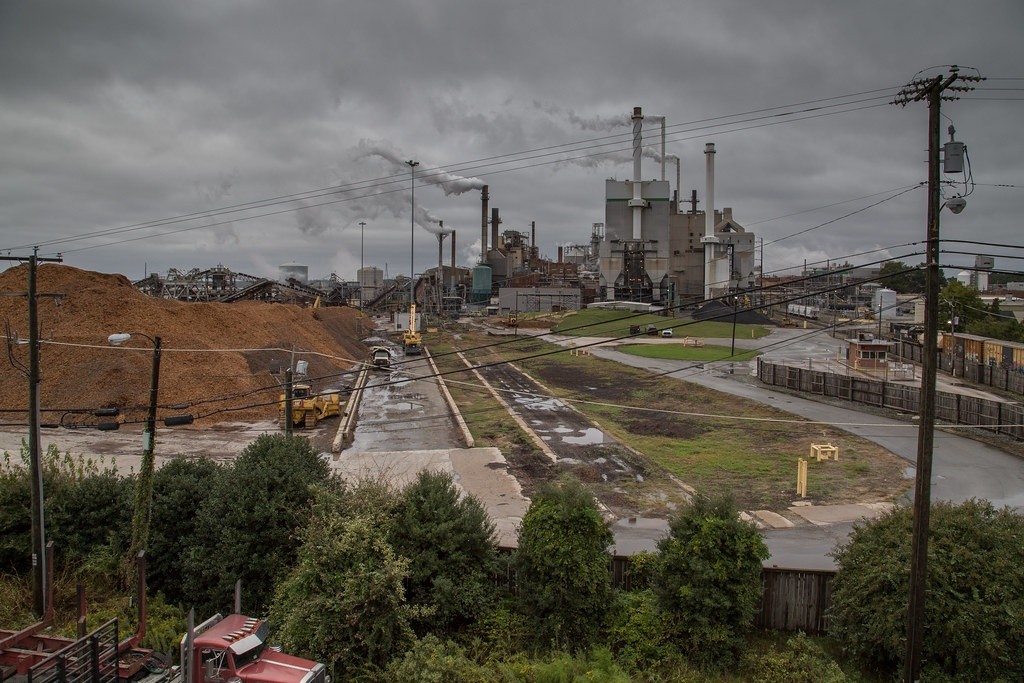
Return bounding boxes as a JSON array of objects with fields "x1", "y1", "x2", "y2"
[
  {"x1": 646, "y1": 323, "x2": 658, "y2": 336},
  {"x1": 662, "y1": 327, "x2": 672, "y2": 338}
]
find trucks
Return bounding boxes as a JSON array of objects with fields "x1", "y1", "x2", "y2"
[
  {"x1": 372, "y1": 345, "x2": 392, "y2": 370},
  {"x1": 0, "y1": 539, "x2": 332, "y2": 683}
]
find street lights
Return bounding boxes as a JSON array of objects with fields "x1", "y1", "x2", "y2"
[
  {"x1": 108, "y1": 330, "x2": 164, "y2": 597},
  {"x1": 902, "y1": 194, "x2": 968, "y2": 683},
  {"x1": 878, "y1": 303, "x2": 883, "y2": 340},
  {"x1": 405, "y1": 160, "x2": 421, "y2": 304},
  {"x1": 358, "y1": 220, "x2": 367, "y2": 312},
  {"x1": 730, "y1": 282, "x2": 741, "y2": 357},
  {"x1": 947, "y1": 319, "x2": 954, "y2": 375}
]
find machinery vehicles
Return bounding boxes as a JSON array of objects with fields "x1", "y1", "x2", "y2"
[
  {"x1": 401, "y1": 304, "x2": 424, "y2": 357},
  {"x1": 278, "y1": 385, "x2": 341, "y2": 429}
]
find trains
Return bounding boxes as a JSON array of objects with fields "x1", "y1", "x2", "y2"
[{"x1": 787, "y1": 303, "x2": 821, "y2": 320}]
